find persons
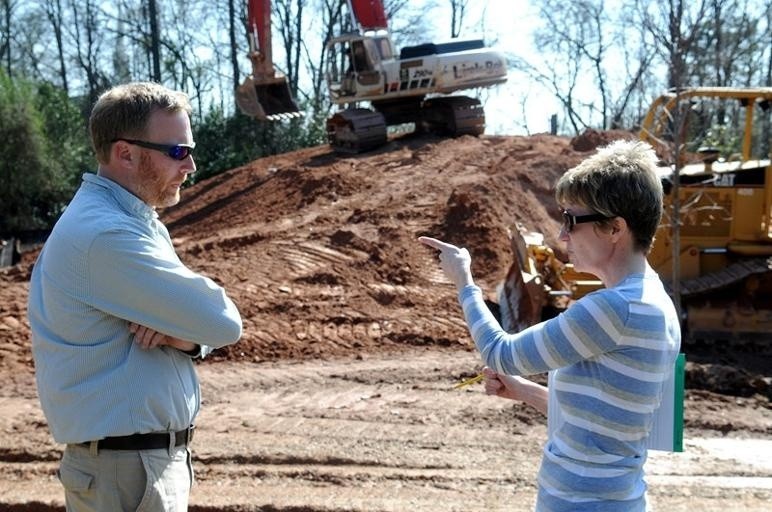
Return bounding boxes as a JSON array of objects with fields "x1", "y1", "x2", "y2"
[
  {"x1": 416, "y1": 140, "x2": 684, "y2": 512},
  {"x1": 26, "y1": 80, "x2": 245, "y2": 510}
]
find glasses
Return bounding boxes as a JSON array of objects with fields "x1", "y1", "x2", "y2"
[
  {"x1": 559, "y1": 210, "x2": 605, "y2": 233},
  {"x1": 110, "y1": 138, "x2": 197, "y2": 160}
]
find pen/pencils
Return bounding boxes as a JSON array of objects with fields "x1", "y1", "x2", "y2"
[{"x1": 454, "y1": 374, "x2": 486, "y2": 390}]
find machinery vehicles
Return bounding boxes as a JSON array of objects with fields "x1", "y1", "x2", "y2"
[
  {"x1": 500, "y1": 87, "x2": 772, "y2": 348},
  {"x1": 234, "y1": 0, "x2": 509, "y2": 154}
]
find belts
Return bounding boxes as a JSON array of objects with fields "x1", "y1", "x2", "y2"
[{"x1": 80, "y1": 426, "x2": 193, "y2": 451}]
